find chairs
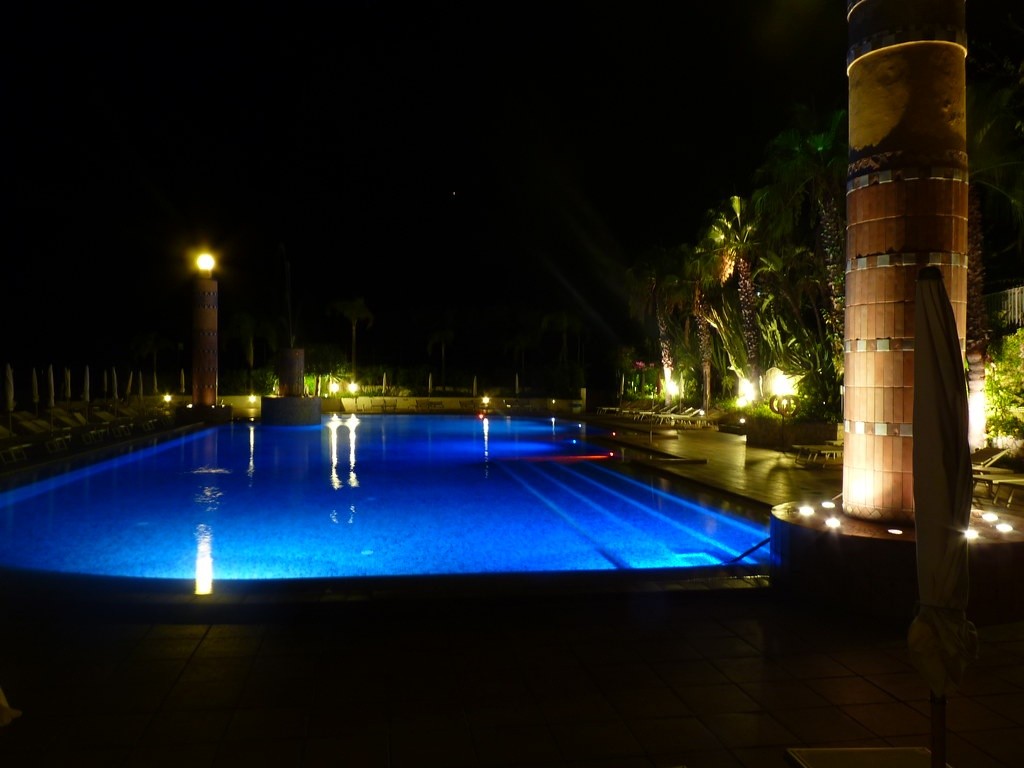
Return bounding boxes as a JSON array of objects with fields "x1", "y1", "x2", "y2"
[
  {"x1": 791, "y1": 421, "x2": 845, "y2": 470},
  {"x1": 0, "y1": 390, "x2": 177, "y2": 465},
  {"x1": 965, "y1": 446, "x2": 1024, "y2": 511},
  {"x1": 597, "y1": 396, "x2": 708, "y2": 427}
]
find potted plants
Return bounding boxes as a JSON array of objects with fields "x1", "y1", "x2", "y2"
[{"x1": 275, "y1": 263, "x2": 304, "y2": 395}]
